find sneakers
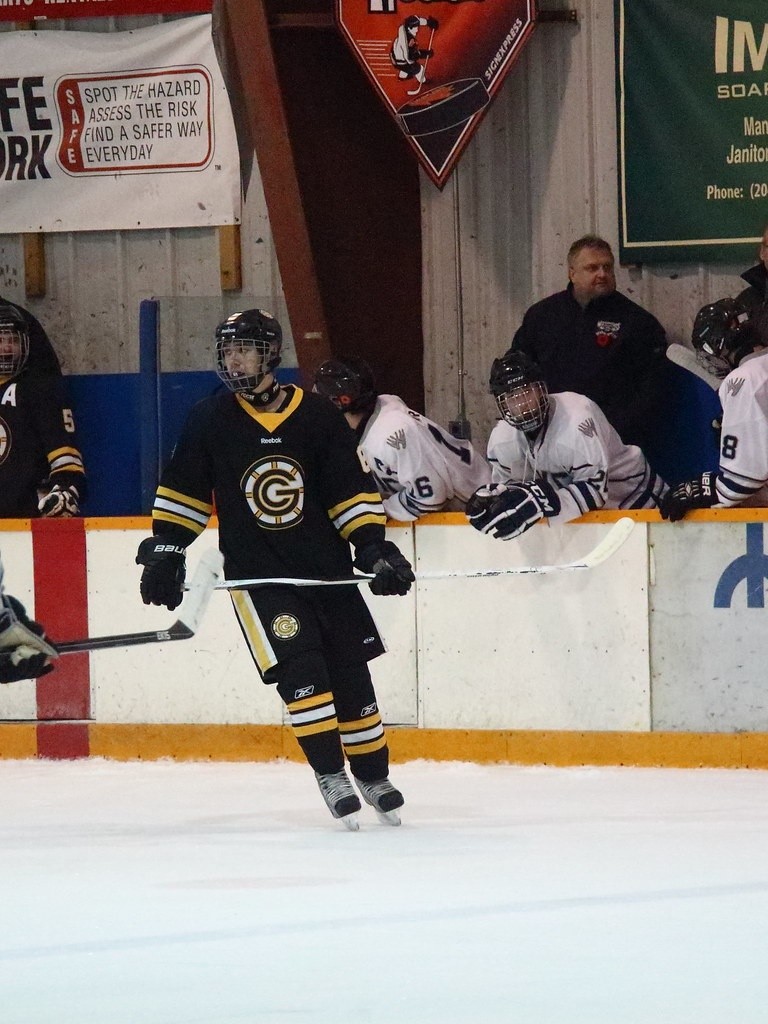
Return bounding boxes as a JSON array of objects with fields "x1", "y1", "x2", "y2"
[
  {"x1": 353, "y1": 776, "x2": 405, "y2": 825},
  {"x1": 314, "y1": 767, "x2": 362, "y2": 832}
]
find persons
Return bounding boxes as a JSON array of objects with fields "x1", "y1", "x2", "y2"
[
  {"x1": 135, "y1": 309, "x2": 417, "y2": 830},
  {"x1": 510, "y1": 228, "x2": 768, "y2": 521},
  {"x1": 464, "y1": 349, "x2": 670, "y2": 541},
  {"x1": 309, "y1": 356, "x2": 492, "y2": 522},
  {"x1": 0, "y1": 308, "x2": 89, "y2": 685}
]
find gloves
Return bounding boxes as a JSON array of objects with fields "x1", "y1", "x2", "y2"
[
  {"x1": 659, "y1": 470, "x2": 718, "y2": 522},
  {"x1": 37, "y1": 483, "x2": 80, "y2": 518},
  {"x1": 351, "y1": 538, "x2": 416, "y2": 595},
  {"x1": 464, "y1": 478, "x2": 561, "y2": 541},
  {"x1": 0, "y1": 594, "x2": 60, "y2": 685},
  {"x1": 135, "y1": 535, "x2": 186, "y2": 611}
]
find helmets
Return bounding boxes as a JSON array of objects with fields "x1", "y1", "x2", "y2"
[
  {"x1": 311, "y1": 354, "x2": 377, "y2": 416},
  {"x1": 489, "y1": 349, "x2": 551, "y2": 434},
  {"x1": 692, "y1": 298, "x2": 762, "y2": 380},
  {"x1": 215, "y1": 309, "x2": 282, "y2": 393},
  {"x1": 1, "y1": 304, "x2": 31, "y2": 377}
]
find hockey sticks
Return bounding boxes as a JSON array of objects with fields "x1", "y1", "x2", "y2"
[
  {"x1": 665, "y1": 342, "x2": 727, "y2": 396},
  {"x1": 54, "y1": 547, "x2": 225, "y2": 655},
  {"x1": 179, "y1": 516, "x2": 637, "y2": 592}
]
